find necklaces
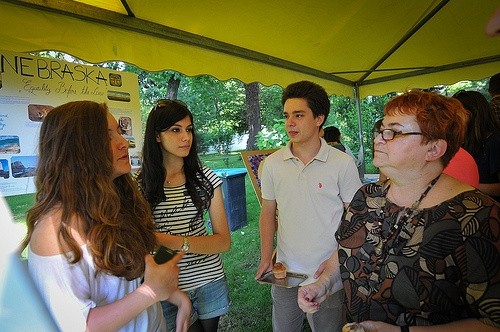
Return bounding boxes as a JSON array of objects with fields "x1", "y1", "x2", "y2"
[{"x1": 377, "y1": 173, "x2": 441, "y2": 243}]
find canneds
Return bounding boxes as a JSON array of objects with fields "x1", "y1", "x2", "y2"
[{"x1": 341, "y1": 322, "x2": 365, "y2": 332}]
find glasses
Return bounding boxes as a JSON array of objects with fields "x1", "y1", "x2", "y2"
[
  {"x1": 154, "y1": 98, "x2": 188, "y2": 111},
  {"x1": 372, "y1": 128, "x2": 422, "y2": 141}
]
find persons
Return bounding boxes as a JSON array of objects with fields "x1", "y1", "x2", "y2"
[
  {"x1": 322, "y1": 126, "x2": 346, "y2": 153},
  {"x1": 255, "y1": 82, "x2": 363, "y2": 332},
  {"x1": 18, "y1": 100, "x2": 192, "y2": 332},
  {"x1": 372, "y1": 73, "x2": 500, "y2": 204},
  {"x1": 135, "y1": 98, "x2": 232, "y2": 332},
  {"x1": 297, "y1": 91, "x2": 500, "y2": 332}
]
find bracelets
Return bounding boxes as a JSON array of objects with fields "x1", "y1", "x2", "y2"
[{"x1": 400, "y1": 325, "x2": 409, "y2": 332}]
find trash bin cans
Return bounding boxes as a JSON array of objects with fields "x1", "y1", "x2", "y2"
[{"x1": 207, "y1": 168, "x2": 248, "y2": 232}]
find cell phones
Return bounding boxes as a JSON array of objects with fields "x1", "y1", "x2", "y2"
[{"x1": 141, "y1": 245, "x2": 175, "y2": 283}]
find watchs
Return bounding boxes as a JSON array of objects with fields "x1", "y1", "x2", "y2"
[{"x1": 176, "y1": 236, "x2": 190, "y2": 255}]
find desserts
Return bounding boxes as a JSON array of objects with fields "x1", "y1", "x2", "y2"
[{"x1": 272, "y1": 263, "x2": 287, "y2": 279}]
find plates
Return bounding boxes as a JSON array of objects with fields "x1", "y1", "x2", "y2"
[{"x1": 256, "y1": 271, "x2": 308, "y2": 289}]
